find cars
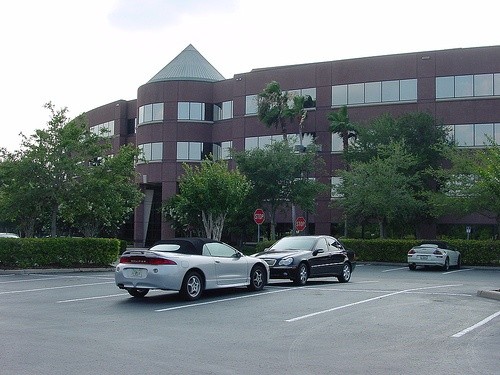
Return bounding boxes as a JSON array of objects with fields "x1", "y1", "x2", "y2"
[
  {"x1": 251, "y1": 235, "x2": 357, "y2": 285},
  {"x1": 407, "y1": 241, "x2": 462, "y2": 272}
]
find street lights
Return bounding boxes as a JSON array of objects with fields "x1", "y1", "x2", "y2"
[{"x1": 466, "y1": 225, "x2": 472, "y2": 240}]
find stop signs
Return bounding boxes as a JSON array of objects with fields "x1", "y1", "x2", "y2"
[
  {"x1": 254, "y1": 208, "x2": 265, "y2": 226},
  {"x1": 295, "y1": 217, "x2": 307, "y2": 231}
]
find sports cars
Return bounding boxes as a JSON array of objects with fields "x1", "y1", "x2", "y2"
[{"x1": 115, "y1": 236, "x2": 270, "y2": 301}]
